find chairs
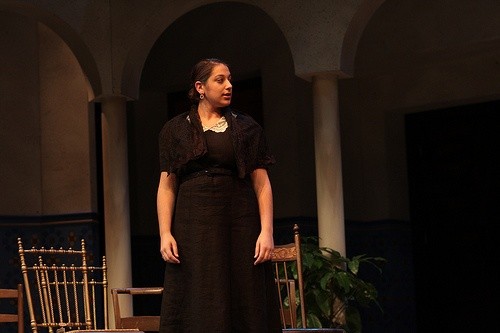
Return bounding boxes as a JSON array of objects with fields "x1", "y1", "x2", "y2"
[
  {"x1": 17, "y1": 238, "x2": 94, "y2": 333},
  {"x1": 111, "y1": 287, "x2": 164, "y2": 332},
  {"x1": 0, "y1": 284, "x2": 25, "y2": 333},
  {"x1": 270, "y1": 222, "x2": 347, "y2": 332},
  {"x1": 38, "y1": 253, "x2": 146, "y2": 333}
]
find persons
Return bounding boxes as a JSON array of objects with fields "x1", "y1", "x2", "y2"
[{"x1": 156, "y1": 54, "x2": 282, "y2": 333}]
